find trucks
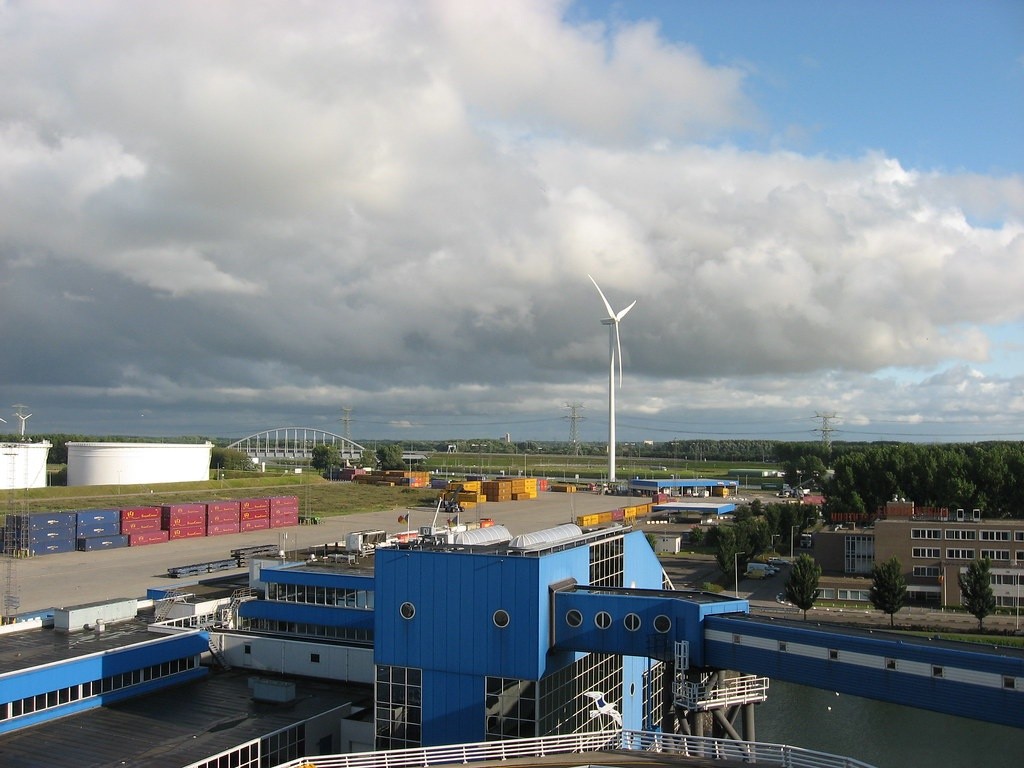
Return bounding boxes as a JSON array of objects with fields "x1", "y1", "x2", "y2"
[
  {"x1": 800, "y1": 533, "x2": 813, "y2": 548},
  {"x1": 650, "y1": 465, "x2": 667, "y2": 472}
]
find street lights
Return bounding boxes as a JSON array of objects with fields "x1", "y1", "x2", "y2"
[
  {"x1": 735, "y1": 551, "x2": 746, "y2": 599},
  {"x1": 771, "y1": 534, "x2": 780, "y2": 546},
  {"x1": 1016, "y1": 573, "x2": 1020, "y2": 631},
  {"x1": 790, "y1": 525, "x2": 800, "y2": 558}
]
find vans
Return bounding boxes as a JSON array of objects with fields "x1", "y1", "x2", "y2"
[{"x1": 743, "y1": 562, "x2": 780, "y2": 580}]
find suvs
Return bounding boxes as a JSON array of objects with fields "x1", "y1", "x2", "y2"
[{"x1": 768, "y1": 557, "x2": 790, "y2": 566}]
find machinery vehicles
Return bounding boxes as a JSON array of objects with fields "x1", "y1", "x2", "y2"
[
  {"x1": 777, "y1": 478, "x2": 816, "y2": 498},
  {"x1": 441, "y1": 484, "x2": 467, "y2": 512}
]
